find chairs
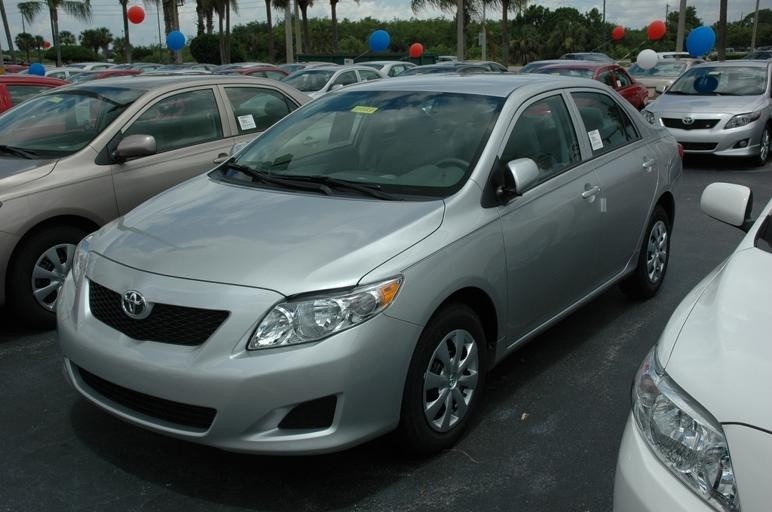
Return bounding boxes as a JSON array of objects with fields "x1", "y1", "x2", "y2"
[
  {"x1": 470, "y1": 113, "x2": 541, "y2": 164},
  {"x1": 376, "y1": 106, "x2": 445, "y2": 176}
]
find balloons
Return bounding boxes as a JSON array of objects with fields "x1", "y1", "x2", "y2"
[
  {"x1": 43, "y1": 40, "x2": 50, "y2": 49},
  {"x1": 166, "y1": 30, "x2": 186, "y2": 52},
  {"x1": 636, "y1": 48, "x2": 659, "y2": 70},
  {"x1": 686, "y1": 24, "x2": 717, "y2": 57},
  {"x1": 127, "y1": 5, "x2": 145, "y2": 25},
  {"x1": 647, "y1": 20, "x2": 667, "y2": 41},
  {"x1": 409, "y1": 42, "x2": 424, "y2": 58},
  {"x1": 368, "y1": 29, "x2": 391, "y2": 52},
  {"x1": 28, "y1": 62, "x2": 45, "y2": 77},
  {"x1": 611, "y1": 25, "x2": 626, "y2": 41}
]
